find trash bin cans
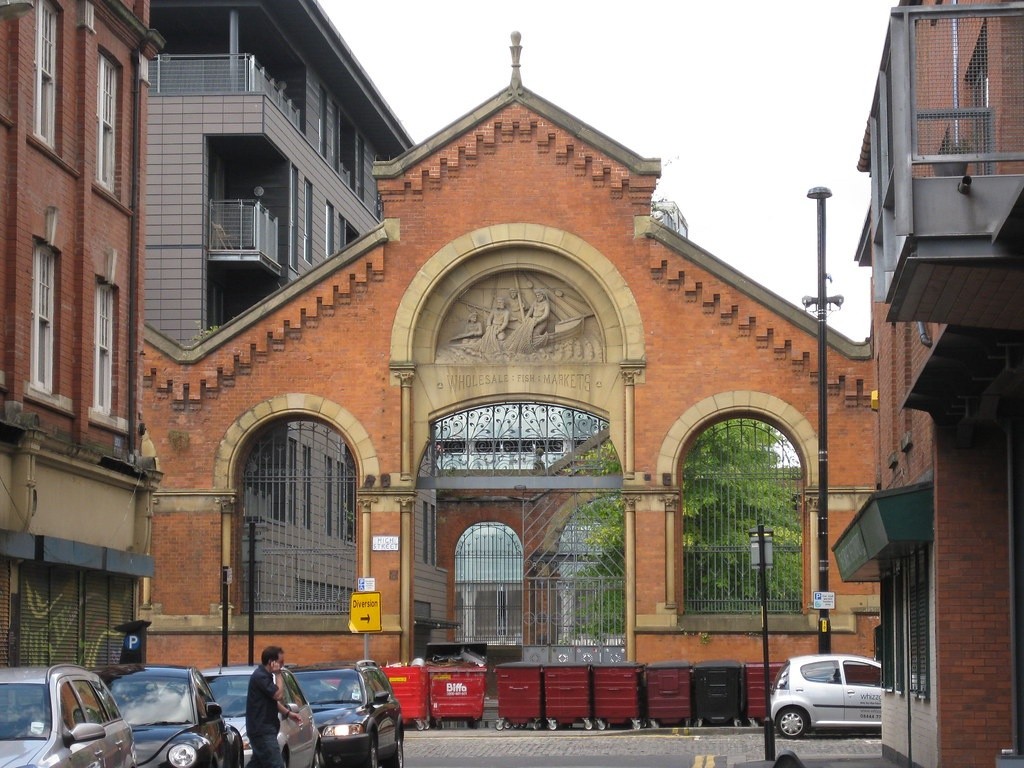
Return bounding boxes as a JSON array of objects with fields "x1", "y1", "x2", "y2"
[
  {"x1": 425, "y1": 641, "x2": 488, "y2": 730},
  {"x1": 590, "y1": 662, "x2": 646, "y2": 731},
  {"x1": 542, "y1": 662, "x2": 593, "y2": 732},
  {"x1": 691, "y1": 659, "x2": 744, "y2": 728},
  {"x1": 379, "y1": 666, "x2": 430, "y2": 731},
  {"x1": 742, "y1": 662, "x2": 785, "y2": 726},
  {"x1": 494, "y1": 661, "x2": 545, "y2": 731},
  {"x1": 642, "y1": 661, "x2": 693, "y2": 728}
]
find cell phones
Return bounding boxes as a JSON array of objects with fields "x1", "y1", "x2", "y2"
[{"x1": 269, "y1": 659, "x2": 273, "y2": 665}]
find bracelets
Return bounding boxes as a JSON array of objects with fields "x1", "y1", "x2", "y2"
[{"x1": 286, "y1": 711, "x2": 290, "y2": 717}]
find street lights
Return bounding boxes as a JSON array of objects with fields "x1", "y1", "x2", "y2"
[
  {"x1": 748, "y1": 522, "x2": 776, "y2": 761},
  {"x1": 802, "y1": 185, "x2": 844, "y2": 655},
  {"x1": 242, "y1": 516, "x2": 266, "y2": 666}
]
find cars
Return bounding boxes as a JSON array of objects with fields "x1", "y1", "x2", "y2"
[{"x1": 770, "y1": 653, "x2": 883, "y2": 740}]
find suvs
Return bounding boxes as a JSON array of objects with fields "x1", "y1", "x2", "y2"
[
  {"x1": 284, "y1": 659, "x2": 404, "y2": 768},
  {"x1": 0, "y1": 662, "x2": 140, "y2": 768},
  {"x1": 196, "y1": 663, "x2": 326, "y2": 768},
  {"x1": 72, "y1": 662, "x2": 245, "y2": 768}
]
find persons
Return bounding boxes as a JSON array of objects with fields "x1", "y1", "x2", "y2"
[
  {"x1": 244, "y1": 646, "x2": 304, "y2": 768},
  {"x1": 462, "y1": 287, "x2": 550, "y2": 343}
]
